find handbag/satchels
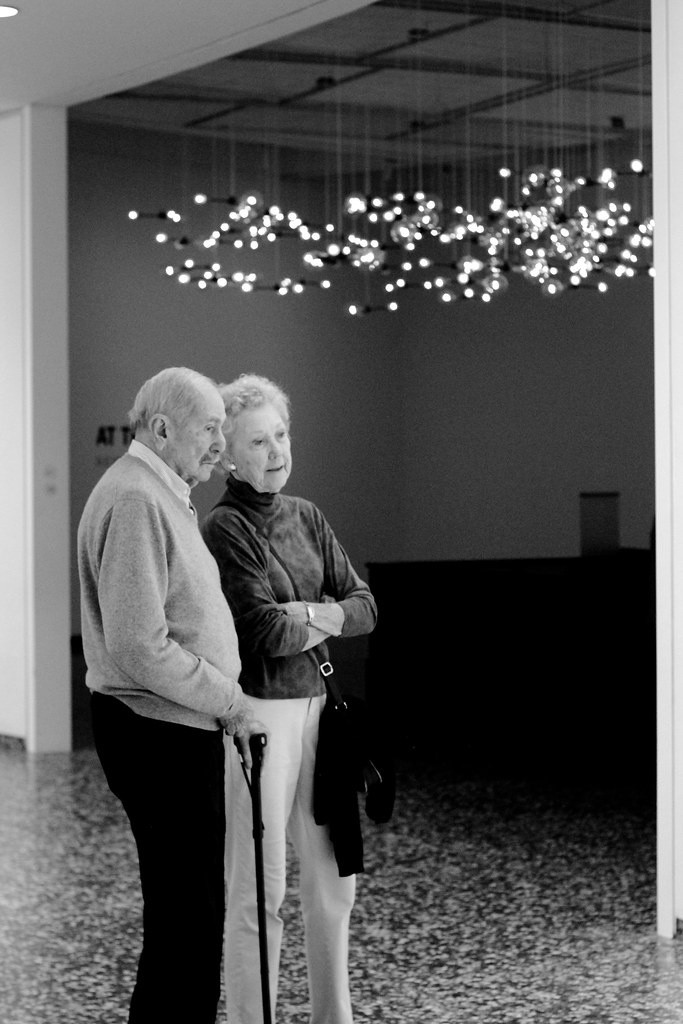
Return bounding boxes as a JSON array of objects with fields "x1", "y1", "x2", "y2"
[{"x1": 339, "y1": 703, "x2": 383, "y2": 793}]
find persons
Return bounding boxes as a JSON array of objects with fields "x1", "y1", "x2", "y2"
[
  {"x1": 200, "y1": 374, "x2": 379, "y2": 1024},
  {"x1": 77, "y1": 365, "x2": 272, "y2": 1024}
]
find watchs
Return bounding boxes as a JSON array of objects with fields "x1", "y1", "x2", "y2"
[{"x1": 302, "y1": 600, "x2": 315, "y2": 626}]
